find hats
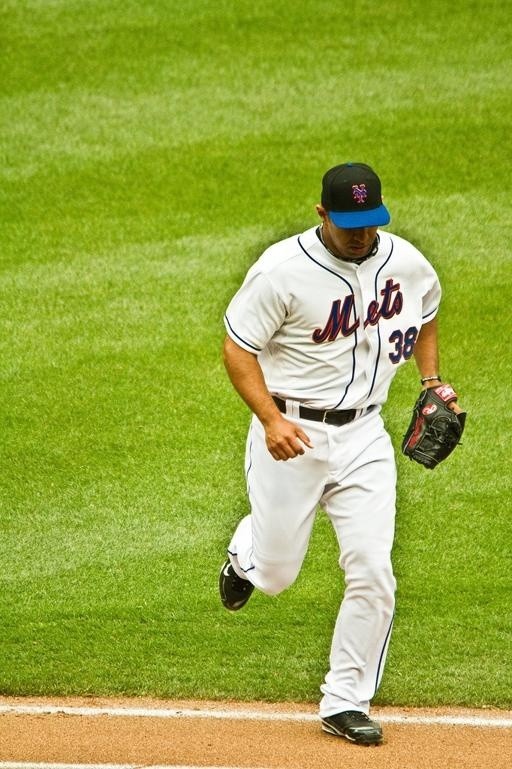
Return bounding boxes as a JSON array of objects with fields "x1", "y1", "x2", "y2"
[{"x1": 320, "y1": 160, "x2": 392, "y2": 230}]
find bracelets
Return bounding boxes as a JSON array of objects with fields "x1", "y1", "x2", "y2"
[{"x1": 419, "y1": 375, "x2": 442, "y2": 385}]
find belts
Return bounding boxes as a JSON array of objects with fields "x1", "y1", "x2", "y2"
[{"x1": 271, "y1": 394, "x2": 376, "y2": 426}]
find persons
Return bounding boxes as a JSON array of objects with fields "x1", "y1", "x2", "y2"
[{"x1": 217, "y1": 161, "x2": 462, "y2": 748}]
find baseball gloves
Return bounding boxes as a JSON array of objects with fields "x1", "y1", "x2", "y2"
[{"x1": 401, "y1": 390, "x2": 468, "y2": 468}]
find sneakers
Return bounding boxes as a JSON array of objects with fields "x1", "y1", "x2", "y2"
[
  {"x1": 320, "y1": 709, "x2": 384, "y2": 745},
  {"x1": 217, "y1": 557, "x2": 256, "y2": 610}
]
